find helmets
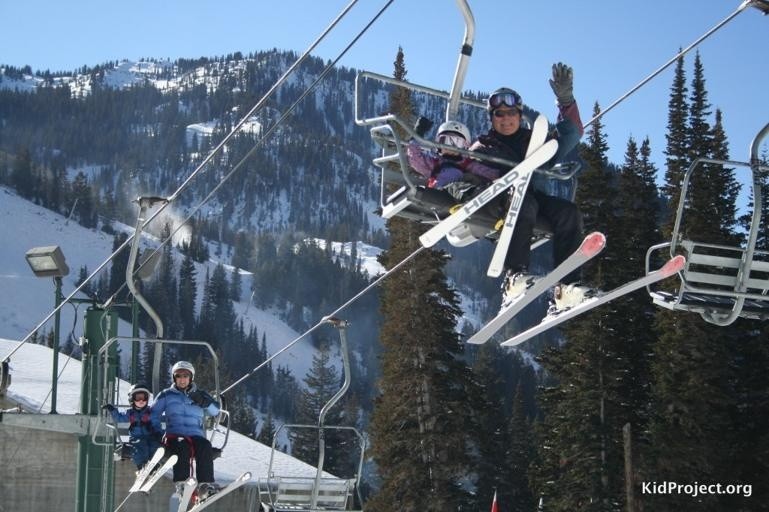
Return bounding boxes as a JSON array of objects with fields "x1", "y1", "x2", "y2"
[
  {"x1": 436, "y1": 120, "x2": 470, "y2": 142},
  {"x1": 129, "y1": 383, "x2": 150, "y2": 405},
  {"x1": 487, "y1": 87, "x2": 523, "y2": 113},
  {"x1": 172, "y1": 361, "x2": 196, "y2": 382}
]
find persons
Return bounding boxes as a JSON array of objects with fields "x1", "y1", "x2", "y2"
[
  {"x1": 408, "y1": 116, "x2": 515, "y2": 218},
  {"x1": 470, "y1": 62, "x2": 606, "y2": 318},
  {"x1": 151, "y1": 360, "x2": 220, "y2": 504},
  {"x1": 101, "y1": 382, "x2": 166, "y2": 476}
]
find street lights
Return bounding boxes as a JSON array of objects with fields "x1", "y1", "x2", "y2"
[{"x1": 25, "y1": 242, "x2": 71, "y2": 415}]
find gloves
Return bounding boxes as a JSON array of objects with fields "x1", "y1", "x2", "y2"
[
  {"x1": 548, "y1": 62, "x2": 575, "y2": 106},
  {"x1": 189, "y1": 389, "x2": 205, "y2": 406},
  {"x1": 414, "y1": 116, "x2": 434, "y2": 137},
  {"x1": 101, "y1": 404, "x2": 114, "y2": 412}
]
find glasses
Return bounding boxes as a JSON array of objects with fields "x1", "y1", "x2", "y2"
[
  {"x1": 175, "y1": 373, "x2": 191, "y2": 379},
  {"x1": 132, "y1": 393, "x2": 148, "y2": 402},
  {"x1": 436, "y1": 134, "x2": 467, "y2": 149},
  {"x1": 489, "y1": 92, "x2": 516, "y2": 108},
  {"x1": 493, "y1": 109, "x2": 518, "y2": 117}
]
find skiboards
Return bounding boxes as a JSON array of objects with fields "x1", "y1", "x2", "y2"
[
  {"x1": 418, "y1": 114, "x2": 558, "y2": 279},
  {"x1": 129, "y1": 447, "x2": 179, "y2": 493},
  {"x1": 465, "y1": 230, "x2": 687, "y2": 348},
  {"x1": 176, "y1": 471, "x2": 252, "y2": 512}
]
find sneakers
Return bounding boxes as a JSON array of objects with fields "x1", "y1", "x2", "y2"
[
  {"x1": 554, "y1": 283, "x2": 603, "y2": 311},
  {"x1": 503, "y1": 273, "x2": 540, "y2": 307},
  {"x1": 175, "y1": 480, "x2": 185, "y2": 502},
  {"x1": 198, "y1": 482, "x2": 220, "y2": 504}
]
